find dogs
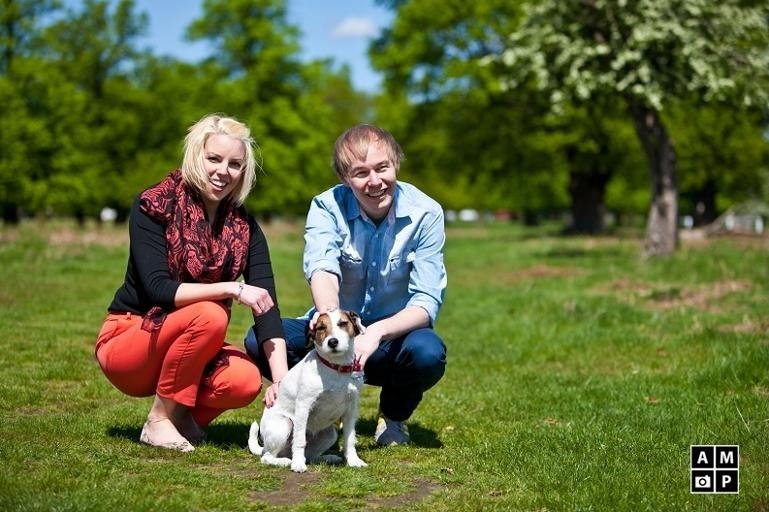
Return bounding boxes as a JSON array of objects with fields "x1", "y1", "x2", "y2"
[{"x1": 248, "y1": 308, "x2": 370, "y2": 474}]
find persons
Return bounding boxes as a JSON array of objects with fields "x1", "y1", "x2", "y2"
[
  {"x1": 91, "y1": 111, "x2": 289, "y2": 451},
  {"x1": 246, "y1": 122, "x2": 449, "y2": 445}
]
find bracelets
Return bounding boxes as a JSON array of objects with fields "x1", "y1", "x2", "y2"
[{"x1": 236, "y1": 281, "x2": 244, "y2": 305}]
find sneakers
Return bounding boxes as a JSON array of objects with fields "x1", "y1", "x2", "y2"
[
  {"x1": 140, "y1": 419, "x2": 206, "y2": 453},
  {"x1": 374, "y1": 412, "x2": 410, "y2": 447}
]
